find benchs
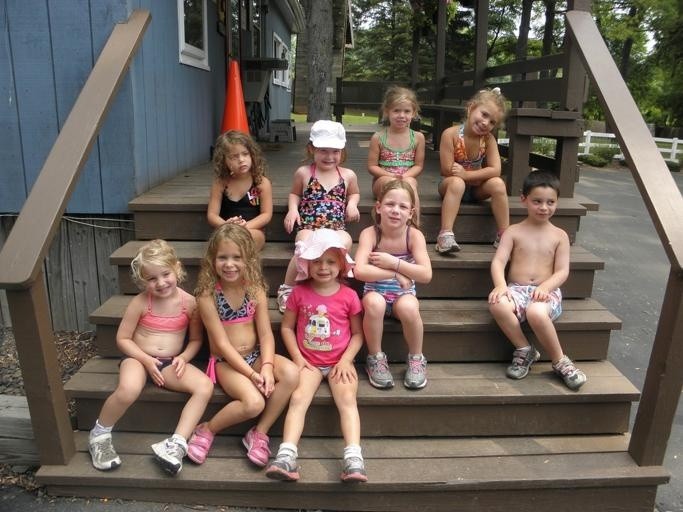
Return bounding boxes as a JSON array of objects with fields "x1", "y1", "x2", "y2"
[{"x1": 330, "y1": 52, "x2": 586, "y2": 199}]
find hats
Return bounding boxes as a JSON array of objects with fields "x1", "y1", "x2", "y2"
[
  {"x1": 310, "y1": 120, "x2": 346, "y2": 150},
  {"x1": 293, "y1": 229, "x2": 354, "y2": 281}
]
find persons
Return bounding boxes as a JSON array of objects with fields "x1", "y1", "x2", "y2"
[
  {"x1": 187, "y1": 221, "x2": 299, "y2": 467},
  {"x1": 368, "y1": 86, "x2": 425, "y2": 228},
  {"x1": 354, "y1": 179, "x2": 433, "y2": 389},
  {"x1": 207, "y1": 129, "x2": 273, "y2": 252},
  {"x1": 435, "y1": 89, "x2": 510, "y2": 253},
  {"x1": 265, "y1": 228, "x2": 368, "y2": 483},
  {"x1": 88, "y1": 238, "x2": 214, "y2": 477},
  {"x1": 488, "y1": 171, "x2": 587, "y2": 389},
  {"x1": 277, "y1": 120, "x2": 361, "y2": 315}
]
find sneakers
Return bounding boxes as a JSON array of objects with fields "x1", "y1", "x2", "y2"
[
  {"x1": 507, "y1": 343, "x2": 540, "y2": 379},
  {"x1": 149, "y1": 423, "x2": 214, "y2": 477},
  {"x1": 277, "y1": 284, "x2": 292, "y2": 313},
  {"x1": 240, "y1": 425, "x2": 301, "y2": 481},
  {"x1": 340, "y1": 451, "x2": 368, "y2": 482},
  {"x1": 365, "y1": 352, "x2": 395, "y2": 389},
  {"x1": 551, "y1": 355, "x2": 587, "y2": 390},
  {"x1": 88, "y1": 423, "x2": 121, "y2": 471},
  {"x1": 404, "y1": 352, "x2": 428, "y2": 389},
  {"x1": 435, "y1": 230, "x2": 462, "y2": 254}
]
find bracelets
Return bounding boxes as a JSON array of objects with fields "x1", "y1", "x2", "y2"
[
  {"x1": 259, "y1": 362, "x2": 274, "y2": 370},
  {"x1": 396, "y1": 259, "x2": 400, "y2": 272},
  {"x1": 249, "y1": 371, "x2": 255, "y2": 378},
  {"x1": 395, "y1": 271, "x2": 396, "y2": 279}
]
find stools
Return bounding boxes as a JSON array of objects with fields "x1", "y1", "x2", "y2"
[{"x1": 268, "y1": 119, "x2": 297, "y2": 143}]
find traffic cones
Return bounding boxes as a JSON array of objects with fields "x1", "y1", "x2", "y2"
[{"x1": 220, "y1": 61, "x2": 250, "y2": 138}]
